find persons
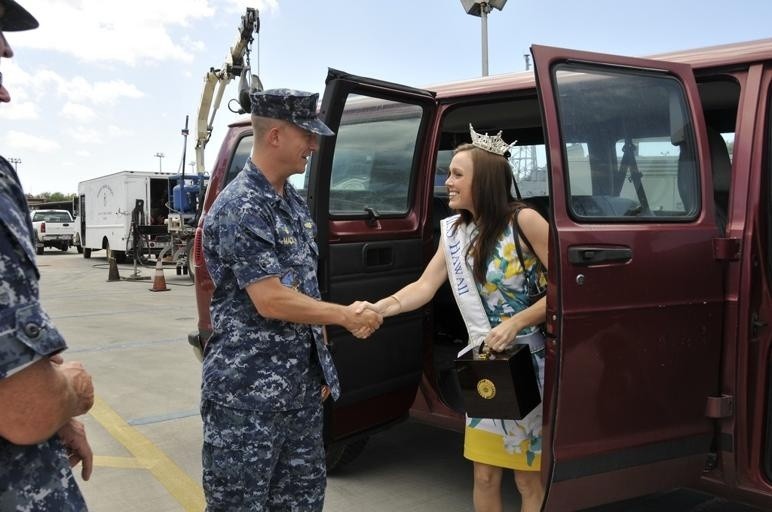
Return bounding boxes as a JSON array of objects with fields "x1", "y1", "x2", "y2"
[
  {"x1": 349, "y1": 144, "x2": 550, "y2": 512},
  {"x1": 199, "y1": 89, "x2": 385, "y2": 512},
  {"x1": 0, "y1": 0, "x2": 94, "y2": 510}
]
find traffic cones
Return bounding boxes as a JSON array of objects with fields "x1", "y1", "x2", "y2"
[
  {"x1": 107, "y1": 256, "x2": 125, "y2": 283},
  {"x1": 148, "y1": 259, "x2": 170, "y2": 292}
]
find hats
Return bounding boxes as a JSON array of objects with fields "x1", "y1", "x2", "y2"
[
  {"x1": 2, "y1": 0, "x2": 38, "y2": 32},
  {"x1": 249, "y1": 88, "x2": 336, "y2": 137}
]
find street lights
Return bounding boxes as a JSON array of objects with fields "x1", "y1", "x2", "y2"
[
  {"x1": 8, "y1": 158, "x2": 23, "y2": 173},
  {"x1": 459, "y1": 0, "x2": 510, "y2": 76},
  {"x1": 154, "y1": 151, "x2": 165, "y2": 171}
]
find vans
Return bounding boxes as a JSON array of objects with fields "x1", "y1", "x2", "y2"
[{"x1": 186, "y1": 40, "x2": 772, "y2": 510}]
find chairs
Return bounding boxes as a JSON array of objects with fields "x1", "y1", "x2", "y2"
[{"x1": 678, "y1": 135, "x2": 732, "y2": 225}]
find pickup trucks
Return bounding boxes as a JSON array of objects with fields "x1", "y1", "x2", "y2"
[{"x1": 29, "y1": 209, "x2": 75, "y2": 255}]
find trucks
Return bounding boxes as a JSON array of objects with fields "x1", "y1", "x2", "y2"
[{"x1": 72, "y1": 170, "x2": 183, "y2": 262}]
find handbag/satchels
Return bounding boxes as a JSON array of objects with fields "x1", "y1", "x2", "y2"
[{"x1": 512, "y1": 211, "x2": 551, "y2": 337}]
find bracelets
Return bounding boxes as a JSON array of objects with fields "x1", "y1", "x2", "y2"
[{"x1": 389, "y1": 295, "x2": 402, "y2": 316}]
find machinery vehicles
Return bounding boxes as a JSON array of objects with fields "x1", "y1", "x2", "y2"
[{"x1": 124, "y1": 6, "x2": 266, "y2": 277}]
